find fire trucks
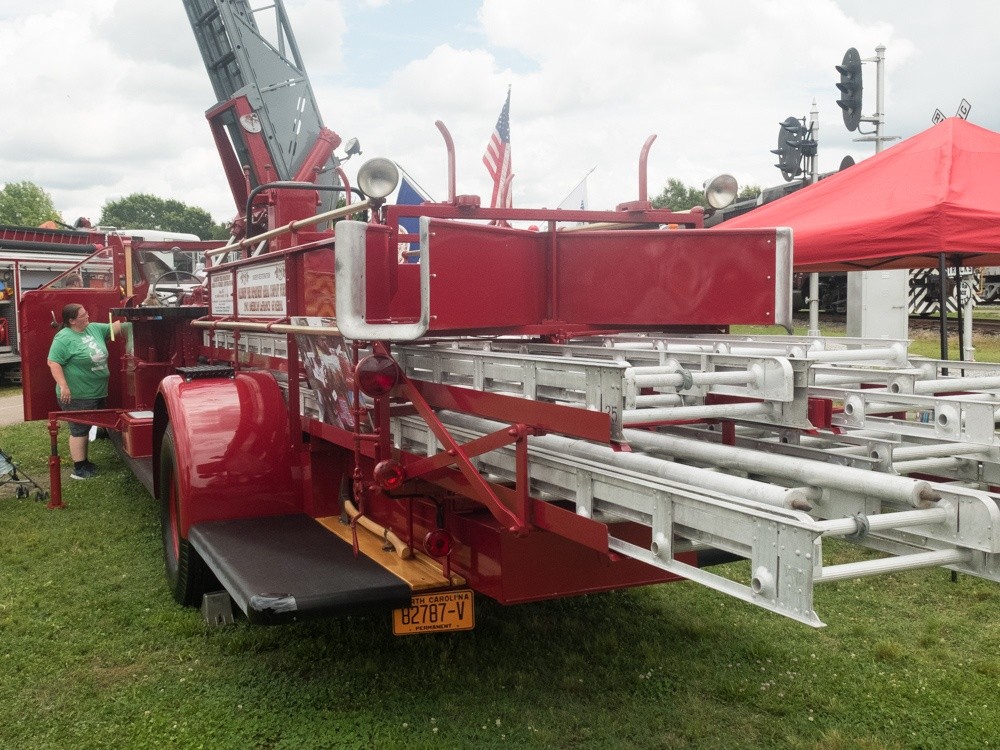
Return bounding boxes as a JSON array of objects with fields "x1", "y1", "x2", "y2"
[{"x1": 1, "y1": 0, "x2": 999, "y2": 640}]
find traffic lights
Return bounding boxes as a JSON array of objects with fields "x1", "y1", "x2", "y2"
[
  {"x1": 834, "y1": 47, "x2": 862, "y2": 132},
  {"x1": 769, "y1": 116, "x2": 803, "y2": 182}
]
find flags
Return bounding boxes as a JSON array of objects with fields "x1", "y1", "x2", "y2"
[{"x1": 482, "y1": 97, "x2": 512, "y2": 227}]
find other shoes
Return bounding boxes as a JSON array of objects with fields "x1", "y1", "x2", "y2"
[
  {"x1": 70, "y1": 468, "x2": 107, "y2": 482},
  {"x1": 87, "y1": 462, "x2": 106, "y2": 473}
]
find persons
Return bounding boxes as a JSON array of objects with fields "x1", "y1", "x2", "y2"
[
  {"x1": 65, "y1": 274, "x2": 83, "y2": 288},
  {"x1": 47, "y1": 305, "x2": 130, "y2": 480},
  {"x1": 143, "y1": 296, "x2": 162, "y2": 320}
]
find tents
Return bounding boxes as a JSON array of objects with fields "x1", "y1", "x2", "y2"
[{"x1": 709, "y1": 116, "x2": 1000, "y2": 377}]
